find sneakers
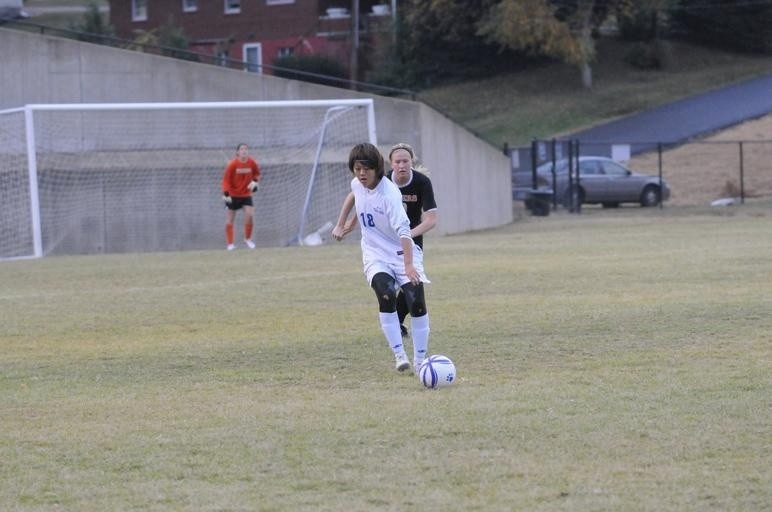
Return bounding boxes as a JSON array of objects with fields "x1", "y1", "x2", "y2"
[
  {"x1": 228, "y1": 243, "x2": 234, "y2": 251},
  {"x1": 396, "y1": 351, "x2": 409, "y2": 370},
  {"x1": 400, "y1": 325, "x2": 408, "y2": 336},
  {"x1": 415, "y1": 362, "x2": 422, "y2": 376},
  {"x1": 244, "y1": 238, "x2": 256, "y2": 249}
]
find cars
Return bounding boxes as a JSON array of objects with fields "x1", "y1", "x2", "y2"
[{"x1": 512, "y1": 156, "x2": 669, "y2": 216}]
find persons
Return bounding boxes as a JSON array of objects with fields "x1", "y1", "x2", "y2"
[
  {"x1": 344, "y1": 144, "x2": 438, "y2": 336},
  {"x1": 333, "y1": 144, "x2": 431, "y2": 376},
  {"x1": 222, "y1": 143, "x2": 260, "y2": 250}
]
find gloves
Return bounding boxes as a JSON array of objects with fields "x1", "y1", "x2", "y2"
[
  {"x1": 223, "y1": 191, "x2": 232, "y2": 204},
  {"x1": 247, "y1": 179, "x2": 259, "y2": 192}
]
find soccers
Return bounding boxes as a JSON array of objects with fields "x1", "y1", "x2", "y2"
[{"x1": 419, "y1": 354, "x2": 457, "y2": 389}]
